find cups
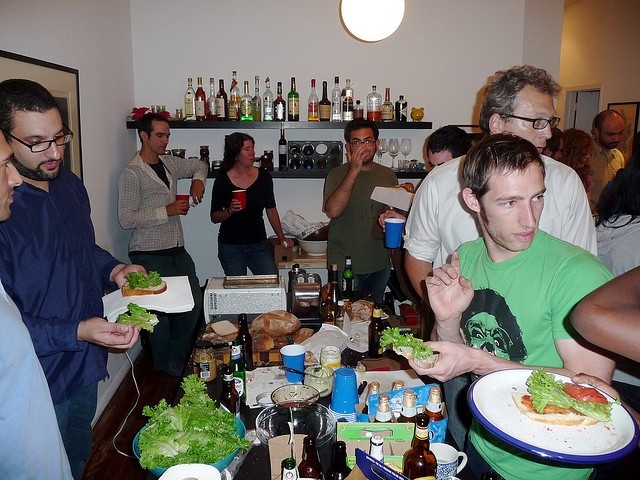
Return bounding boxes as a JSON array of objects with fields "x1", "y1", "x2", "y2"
[
  {"x1": 428, "y1": 443, "x2": 468, "y2": 480},
  {"x1": 302, "y1": 365, "x2": 334, "y2": 398},
  {"x1": 231, "y1": 189, "x2": 247, "y2": 211},
  {"x1": 384, "y1": 218, "x2": 406, "y2": 250},
  {"x1": 328, "y1": 368, "x2": 360, "y2": 422},
  {"x1": 279, "y1": 344, "x2": 307, "y2": 384},
  {"x1": 175, "y1": 193, "x2": 191, "y2": 216}
]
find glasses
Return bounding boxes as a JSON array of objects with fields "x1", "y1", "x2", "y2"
[
  {"x1": 1, "y1": 123, "x2": 74, "y2": 152},
  {"x1": 346, "y1": 139, "x2": 378, "y2": 147},
  {"x1": 502, "y1": 114, "x2": 561, "y2": 129}
]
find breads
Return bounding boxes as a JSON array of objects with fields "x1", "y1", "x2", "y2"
[
  {"x1": 209, "y1": 318, "x2": 239, "y2": 337},
  {"x1": 247, "y1": 309, "x2": 314, "y2": 367},
  {"x1": 343, "y1": 298, "x2": 375, "y2": 324}
]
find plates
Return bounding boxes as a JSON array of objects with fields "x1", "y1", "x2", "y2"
[{"x1": 467, "y1": 369, "x2": 640, "y2": 466}]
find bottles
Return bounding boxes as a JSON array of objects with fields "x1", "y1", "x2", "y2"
[
  {"x1": 395, "y1": 95, "x2": 408, "y2": 122},
  {"x1": 278, "y1": 128, "x2": 288, "y2": 173},
  {"x1": 400, "y1": 412, "x2": 425, "y2": 479},
  {"x1": 361, "y1": 381, "x2": 382, "y2": 417},
  {"x1": 227, "y1": 70, "x2": 242, "y2": 122},
  {"x1": 322, "y1": 296, "x2": 335, "y2": 326},
  {"x1": 280, "y1": 457, "x2": 297, "y2": 480},
  {"x1": 262, "y1": 155, "x2": 269, "y2": 170},
  {"x1": 194, "y1": 76, "x2": 207, "y2": 124},
  {"x1": 240, "y1": 80, "x2": 254, "y2": 122},
  {"x1": 342, "y1": 255, "x2": 356, "y2": 293},
  {"x1": 325, "y1": 263, "x2": 342, "y2": 318},
  {"x1": 264, "y1": 149, "x2": 274, "y2": 170},
  {"x1": 372, "y1": 393, "x2": 396, "y2": 423},
  {"x1": 273, "y1": 81, "x2": 286, "y2": 122},
  {"x1": 320, "y1": 346, "x2": 341, "y2": 372},
  {"x1": 215, "y1": 78, "x2": 228, "y2": 121},
  {"x1": 317, "y1": 80, "x2": 331, "y2": 121},
  {"x1": 251, "y1": 74, "x2": 261, "y2": 122},
  {"x1": 205, "y1": 77, "x2": 216, "y2": 121},
  {"x1": 335, "y1": 300, "x2": 347, "y2": 332},
  {"x1": 353, "y1": 100, "x2": 364, "y2": 119},
  {"x1": 184, "y1": 77, "x2": 196, "y2": 122},
  {"x1": 380, "y1": 88, "x2": 394, "y2": 123},
  {"x1": 235, "y1": 313, "x2": 253, "y2": 372},
  {"x1": 423, "y1": 386, "x2": 445, "y2": 425},
  {"x1": 307, "y1": 78, "x2": 320, "y2": 122},
  {"x1": 227, "y1": 338, "x2": 248, "y2": 405},
  {"x1": 331, "y1": 77, "x2": 343, "y2": 123},
  {"x1": 287, "y1": 77, "x2": 300, "y2": 122},
  {"x1": 297, "y1": 433, "x2": 324, "y2": 479},
  {"x1": 369, "y1": 434, "x2": 384, "y2": 468},
  {"x1": 219, "y1": 364, "x2": 241, "y2": 425},
  {"x1": 340, "y1": 78, "x2": 354, "y2": 123},
  {"x1": 367, "y1": 85, "x2": 383, "y2": 123},
  {"x1": 323, "y1": 440, "x2": 354, "y2": 480},
  {"x1": 396, "y1": 390, "x2": 418, "y2": 423},
  {"x1": 263, "y1": 77, "x2": 275, "y2": 122},
  {"x1": 200, "y1": 146, "x2": 209, "y2": 156},
  {"x1": 367, "y1": 301, "x2": 383, "y2": 359},
  {"x1": 391, "y1": 379, "x2": 405, "y2": 393}
]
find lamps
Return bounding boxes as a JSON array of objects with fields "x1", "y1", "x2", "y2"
[{"x1": 340, "y1": 0, "x2": 405, "y2": 43}]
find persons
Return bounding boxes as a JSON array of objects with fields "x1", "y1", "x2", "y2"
[
  {"x1": 0, "y1": 79, "x2": 148, "y2": 480},
  {"x1": 408, "y1": 133, "x2": 616, "y2": 480},
  {"x1": 378, "y1": 125, "x2": 473, "y2": 248},
  {"x1": 570, "y1": 266, "x2": 639, "y2": 362},
  {"x1": 403, "y1": 65, "x2": 598, "y2": 302},
  {"x1": 322, "y1": 119, "x2": 414, "y2": 307},
  {"x1": 592, "y1": 132, "x2": 640, "y2": 277},
  {"x1": 542, "y1": 127, "x2": 564, "y2": 162},
  {"x1": 118, "y1": 112, "x2": 208, "y2": 377},
  {"x1": 590, "y1": 109, "x2": 625, "y2": 211},
  {"x1": 0, "y1": 130, "x2": 75, "y2": 480},
  {"x1": 561, "y1": 127, "x2": 593, "y2": 210},
  {"x1": 210, "y1": 132, "x2": 294, "y2": 276}
]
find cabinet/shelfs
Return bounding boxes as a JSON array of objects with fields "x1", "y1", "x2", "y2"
[{"x1": 126, "y1": 120, "x2": 435, "y2": 178}]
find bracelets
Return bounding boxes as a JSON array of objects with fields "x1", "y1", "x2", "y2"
[{"x1": 227, "y1": 207, "x2": 233, "y2": 214}]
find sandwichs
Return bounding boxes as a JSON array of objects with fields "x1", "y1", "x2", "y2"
[
  {"x1": 120, "y1": 269, "x2": 168, "y2": 298},
  {"x1": 379, "y1": 326, "x2": 440, "y2": 370},
  {"x1": 512, "y1": 370, "x2": 613, "y2": 427}
]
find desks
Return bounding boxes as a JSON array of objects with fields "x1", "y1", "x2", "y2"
[{"x1": 275, "y1": 245, "x2": 329, "y2": 269}]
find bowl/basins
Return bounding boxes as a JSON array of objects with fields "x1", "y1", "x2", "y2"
[
  {"x1": 131, "y1": 405, "x2": 246, "y2": 479},
  {"x1": 254, "y1": 399, "x2": 337, "y2": 456},
  {"x1": 271, "y1": 383, "x2": 320, "y2": 408},
  {"x1": 302, "y1": 224, "x2": 329, "y2": 241},
  {"x1": 255, "y1": 390, "x2": 276, "y2": 408},
  {"x1": 295, "y1": 237, "x2": 328, "y2": 257}
]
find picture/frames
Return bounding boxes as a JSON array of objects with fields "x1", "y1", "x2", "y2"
[
  {"x1": 0, "y1": 51, "x2": 83, "y2": 187},
  {"x1": 607, "y1": 102, "x2": 640, "y2": 137}
]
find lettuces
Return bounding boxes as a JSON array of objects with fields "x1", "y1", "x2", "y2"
[
  {"x1": 135, "y1": 372, "x2": 251, "y2": 472},
  {"x1": 113, "y1": 302, "x2": 159, "y2": 333}
]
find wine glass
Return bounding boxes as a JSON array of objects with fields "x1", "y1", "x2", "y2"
[
  {"x1": 376, "y1": 136, "x2": 388, "y2": 167},
  {"x1": 388, "y1": 137, "x2": 400, "y2": 172},
  {"x1": 399, "y1": 138, "x2": 413, "y2": 172}
]
